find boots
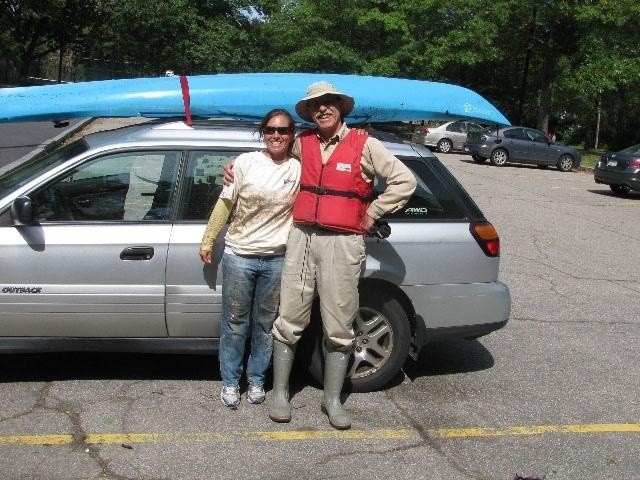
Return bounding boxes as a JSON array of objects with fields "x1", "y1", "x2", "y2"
[
  {"x1": 321, "y1": 340, "x2": 354, "y2": 430},
  {"x1": 269, "y1": 333, "x2": 299, "y2": 423}
]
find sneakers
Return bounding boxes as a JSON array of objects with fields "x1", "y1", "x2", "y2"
[
  {"x1": 222, "y1": 384, "x2": 240, "y2": 408},
  {"x1": 247, "y1": 383, "x2": 265, "y2": 404}
]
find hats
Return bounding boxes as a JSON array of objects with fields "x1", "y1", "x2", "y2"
[{"x1": 294, "y1": 81, "x2": 354, "y2": 122}]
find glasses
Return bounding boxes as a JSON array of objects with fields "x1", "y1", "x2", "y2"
[{"x1": 263, "y1": 124, "x2": 295, "y2": 135}]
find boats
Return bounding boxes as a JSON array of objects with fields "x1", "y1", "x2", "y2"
[{"x1": 1, "y1": 71, "x2": 511, "y2": 127}]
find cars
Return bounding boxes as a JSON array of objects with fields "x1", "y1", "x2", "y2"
[
  {"x1": 0, "y1": 119, "x2": 511, "y2": 393},
  {"x1": 463, "y1": 127, "x2": 581, "y2": 172},
  {"x1": 412, "y1": 123, "x2": 491, "y2": 153},
  {"x1": 594, "y1": 144, "x2": 640, "y2": 194}
]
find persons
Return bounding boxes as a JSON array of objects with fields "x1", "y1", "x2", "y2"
[
  {"x1": 270, "y1": 81, "x2": 417, "y2": 428},
  {"x1": 200, "y1": 109, "x2": 304, "y2": 408}
]
